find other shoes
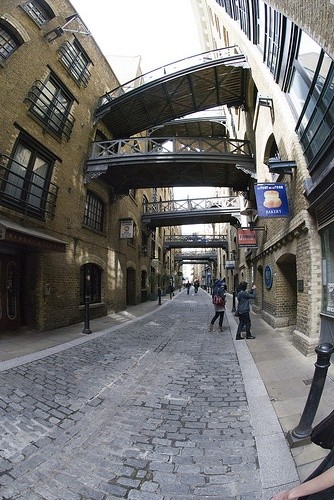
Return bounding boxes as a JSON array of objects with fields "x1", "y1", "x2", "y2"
[
  {"x1": 236, "y1": 337, "x2": 244, "y2": 340},
  {"x1": 210, "y1": 324, "x2": 214, "y2": 331},
  {"x1": 246, "y1": 336, "x2": 255, "y2": 339},
  {"x1": 219, "y1": 326, "x2": 224, "y2": 332}
]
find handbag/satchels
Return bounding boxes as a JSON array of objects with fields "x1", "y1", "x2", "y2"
[
  {"x1": 213, "y1": 288, "x2": 225, "y2": 307},
  {"x1": 234, "y1": 309, "x2": 239, "y2": 317}
]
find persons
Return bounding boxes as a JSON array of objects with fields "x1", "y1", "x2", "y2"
[
  {"x1": 186, "y1": 281, "x2": 191, "y2": 295},
  {"x1": 235, "y1": 283, "x2": 243, "y2": 317},
  {"x1": 269, "y1": 410, "x2": 334, "y2": 500},
  {"x1": 235, "y1": 282, "x2": 257, "y2": 341},
  {"x1": 194, "y1": 279, "x2": 200, "y2": 292},
  {"x1": 208, "y1": 280, "x2": 227, "y2": 333}
]
